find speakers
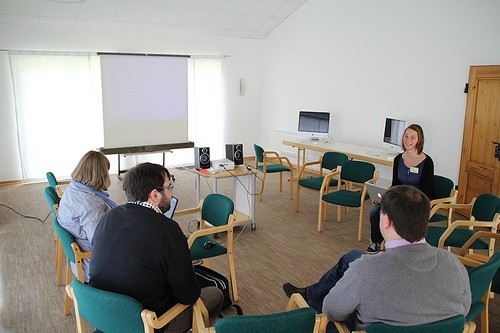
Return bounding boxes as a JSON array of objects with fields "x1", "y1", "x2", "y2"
[
  {"x1": 194, "y1": 147, "x2": 210, "y2": 169},
  {"x1": 226, "y1": 144, "x2": 243, "y2": 166}
]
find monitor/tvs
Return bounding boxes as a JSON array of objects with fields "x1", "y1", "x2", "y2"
[
  {"x1": 298, "y1": 112, "x2": 330, "y2": 133},
  {"x1": 384, "y1": 118, "x2": 406, "y2": 147},
  {"x1": 163, "y1": 196, "x2": 178, "y2": 218}
]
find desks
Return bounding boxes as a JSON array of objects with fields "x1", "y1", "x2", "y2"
[{"x1": 283, "y1": 137, "x2": 399, "y2": 181}]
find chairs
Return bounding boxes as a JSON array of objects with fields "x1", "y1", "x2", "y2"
[{"x1": 45, "y1": 144, "x2": 500, "y2": 333}]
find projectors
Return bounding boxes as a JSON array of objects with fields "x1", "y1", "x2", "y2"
[{"x1": 211, "y1": 158, "x2": 235, "y2": 171}]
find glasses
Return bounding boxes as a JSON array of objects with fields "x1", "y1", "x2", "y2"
[{"x1": 157, "y1": 184, "x2": 174, "y2": 191}]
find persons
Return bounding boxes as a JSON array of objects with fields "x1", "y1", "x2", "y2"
[
  {"x1": 283, "y1": 185, "x2": 471, "y2": 333},
  {"x1": 88, "y1": 162, "x2": 224, "y2": 333},
  {"x1": 57, "y1": 150, "x2": 118, "y2": 283},
  {"x1": 367, "y1": 124, "x2": 434, "y2": 253}
]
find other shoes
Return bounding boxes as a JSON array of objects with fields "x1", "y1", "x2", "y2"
[{"x1": 282, "y1": 283, "x2": 309, "y2": 302}]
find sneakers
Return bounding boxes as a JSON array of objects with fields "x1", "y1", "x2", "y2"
[{"x1": 367, "y1": 242, "x2": 380, "y2": 254}]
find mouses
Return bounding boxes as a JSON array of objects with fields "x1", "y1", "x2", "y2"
[{"x1": 204, "y1": 241, "x2": 216, "y2": 249}]
